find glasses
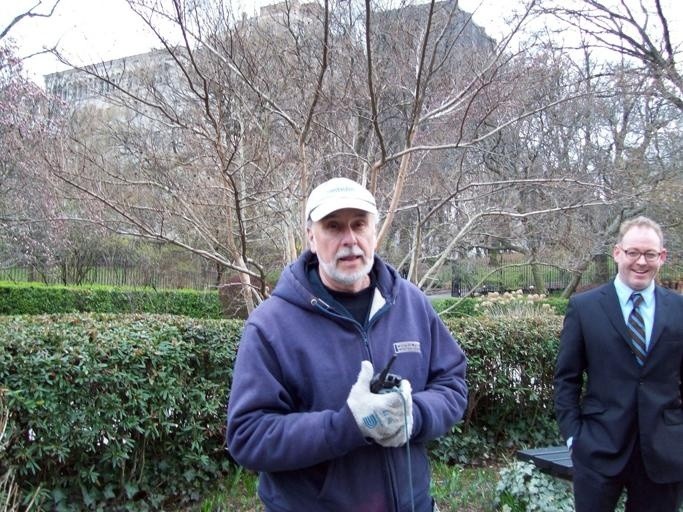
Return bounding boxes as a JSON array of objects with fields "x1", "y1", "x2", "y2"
[{"x1": 618, "y1": 246, "x2": 664, "y2": 263}]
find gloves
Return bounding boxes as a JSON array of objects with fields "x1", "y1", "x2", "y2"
[
  {"x1": 344, "y1": 359, "x2": 412, "y2": 443},
  {"x1": 369, "y1": 415, "x2": 413, "y2": 451}
]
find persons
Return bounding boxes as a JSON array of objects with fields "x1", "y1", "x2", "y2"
[
  {"x1": 225, "y1": 176, "x2": 471, "y2": 512},
  {"x1": 547, "y1": 214, "x2": 683, "y2": 511}
]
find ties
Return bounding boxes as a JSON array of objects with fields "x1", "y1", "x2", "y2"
[{"x1": 626, "y1": 293, "x2": 649, "y2": 367}]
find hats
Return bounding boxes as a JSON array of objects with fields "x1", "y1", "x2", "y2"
[{"x1": 303, "y1": 175, "x2": 379, "y2": 225}]
